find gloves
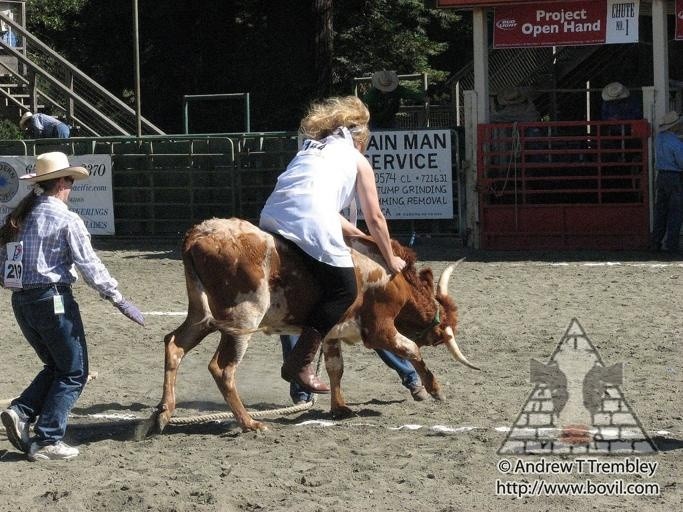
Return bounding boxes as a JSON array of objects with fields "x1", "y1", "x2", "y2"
[{"x1": 112, "y1": 297, "x2": 148, "y2": 328}]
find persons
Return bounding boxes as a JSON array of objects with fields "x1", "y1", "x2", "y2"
[
  {"x1": 497, "y1": 88, "x2": 546, "y2": 202},
  {"x1": 600, "y1": 81, "x2": 641, "y2": 202},
  {"x1": 649, "y1": 108, "x2": 682, "y2": 256},
  {"x1": 18, "y1": 110, "x2": 70, "y2": 145},
  {"x1": 364, "y1": 70, "x2": 430, "y2": 132},
  {"x1": 277, "y1": 196, "x2": 430, "y2": 407},
  {"x1": 258, "y1": 93, "x2": 408, "y2": 396},
  {"x1": 0, "y1": 151, "x2": 155, "y2": 466}
]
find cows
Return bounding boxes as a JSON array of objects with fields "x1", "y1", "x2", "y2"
[{"x1": 151, "y1": 217, "x2": 481, "y2": 436}]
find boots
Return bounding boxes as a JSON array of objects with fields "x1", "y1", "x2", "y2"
[{"x1": 279, "y1": 325, "x2": 332, "y2": 395}]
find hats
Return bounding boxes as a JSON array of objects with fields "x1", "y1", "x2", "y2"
[
  {"x1": 19, "y1": 111, "x2": 33, "y2": 131},
  {"x1": 600, "y1": 81, "x2": 630, "y2": 102},
  {"x1": 373, "y1": 69, "x2": 400, "y2": 92},
  {"x1": 16, "y1": 150, "x2": 91, "y2": 183},
  {"x1": 496, "y1": 86, "x2": 530, "y2": 106},
  {"x1": 657, "y1": 109, "x2": 683, "y2": 134}
]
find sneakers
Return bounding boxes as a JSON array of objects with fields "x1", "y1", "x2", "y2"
[
  {"x1": 0, "y1": 404, "x2": 35, "y2": 454},
  {"x1": 24, "y1": 441, "x2": 81, "y2": 465},
  {"x1": 410, "y1": 384, "x2": 428, "y2": 402}
]
